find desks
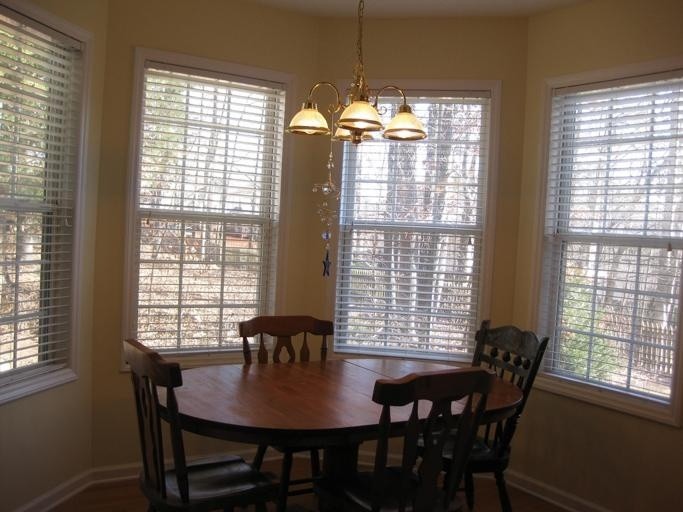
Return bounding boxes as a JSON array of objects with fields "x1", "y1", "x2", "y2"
[{"x1": 156, "y1": 357, "x2": 525, "y2": 512}]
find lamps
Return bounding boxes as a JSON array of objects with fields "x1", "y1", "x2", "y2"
[{"x1": 283, "y1": 0, "x2": 429, "y2": 143}]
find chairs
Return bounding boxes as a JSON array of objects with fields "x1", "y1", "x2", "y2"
[
  {"x1": 313, "y1": 367, "x2": 501, "y2": 509},
  {"x1": 406, "y1": 319, "x2": 551, "y2": 511},
  {"x1": 236, "y1": 314, "x2": 334, "y2": 479},
  {"x1": 121, "y1": 335, "x2": 288, "y2": 511}
]
